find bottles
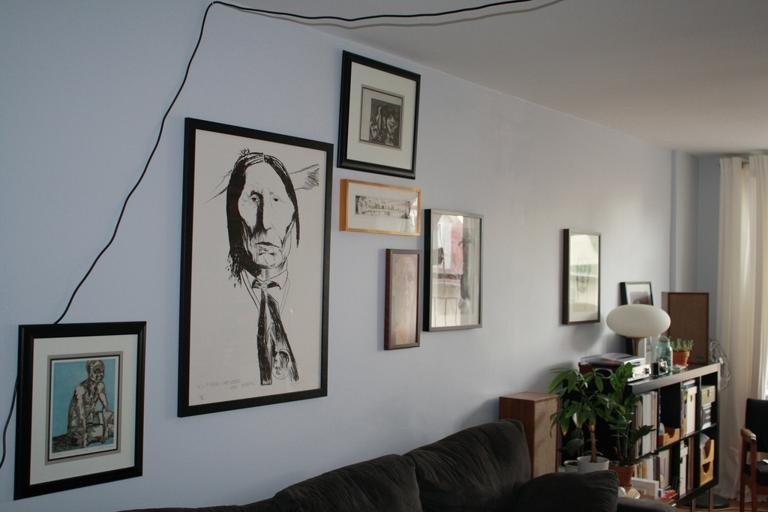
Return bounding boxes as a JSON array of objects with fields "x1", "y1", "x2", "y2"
[{"x1": 655, "y1": 336, "x2": 675, "y2": 371}]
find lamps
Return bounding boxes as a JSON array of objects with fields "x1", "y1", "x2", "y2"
[{"x1": 605, "y1": 306, "x2": 672, "y2": 358}]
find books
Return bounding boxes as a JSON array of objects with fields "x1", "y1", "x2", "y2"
[{"x1": 629, "y1": 377, "x2": 717, "y2": 502}]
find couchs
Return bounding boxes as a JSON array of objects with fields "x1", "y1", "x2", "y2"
[{"x1": 133, "y1": 416, "x2": 679, "y2": 512}]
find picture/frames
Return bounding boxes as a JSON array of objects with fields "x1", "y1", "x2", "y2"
[
  {"x1": 340, "y1": 177, "x2": 425, "y2": 236},
  {"x1": 337, "y1": 48, "x2": 421, "y2": 181},
  {"x1": 177, "y1": 118, "x2": 333, "y2": 417},
  {"x1": 15, "y1": 320, "x2": 146, "y2": 500},
  {"x1": 422, "y1": 207, "x2": 483, "y2": 333},
  {"x1": 562, "y1": 230, "x2": 600, "y2": 327},
  {"x1": 664, "y1": 292, "x2": 710, "y2": 363},
  {"x1": 384, "y1": 248, "x2": 420, "y2": 350},
  {"x1": 621, "y1": 282, "x2": 656, "y2": 364}
]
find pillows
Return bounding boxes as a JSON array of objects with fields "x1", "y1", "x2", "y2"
[{"x1": 514, "y1": 472, "x2": 619, "y2": 511}]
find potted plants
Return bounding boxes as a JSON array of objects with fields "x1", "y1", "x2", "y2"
[
  {"x1": 548, "y1": 362, "x2": 645, "y2": 474},
  {"x1": 605, "y1": 400, "x2": 661, "y2": 491},
  {"x1": 668, "y1": 336, "x2": 694, "y2": 368}
]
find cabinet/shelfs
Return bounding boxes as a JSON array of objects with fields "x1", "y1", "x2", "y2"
[
  {"x1": 559, "y1": 363, "x2": 725, "y2": 512},
  {"x1": 499, "y1": 390, "x2": 562, "y2": 478}
]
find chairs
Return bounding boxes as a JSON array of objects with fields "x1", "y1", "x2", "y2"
[{"x1": 738, "y1": 397, "x2": 768, "y2": 512}]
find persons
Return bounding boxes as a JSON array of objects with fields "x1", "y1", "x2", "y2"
[
  {"x1": 67, "y1": 359, "x2": 114, "y2": 448},
  {"x1": 216, "y1": 149, "x2": 315, "y2": 389}
]
